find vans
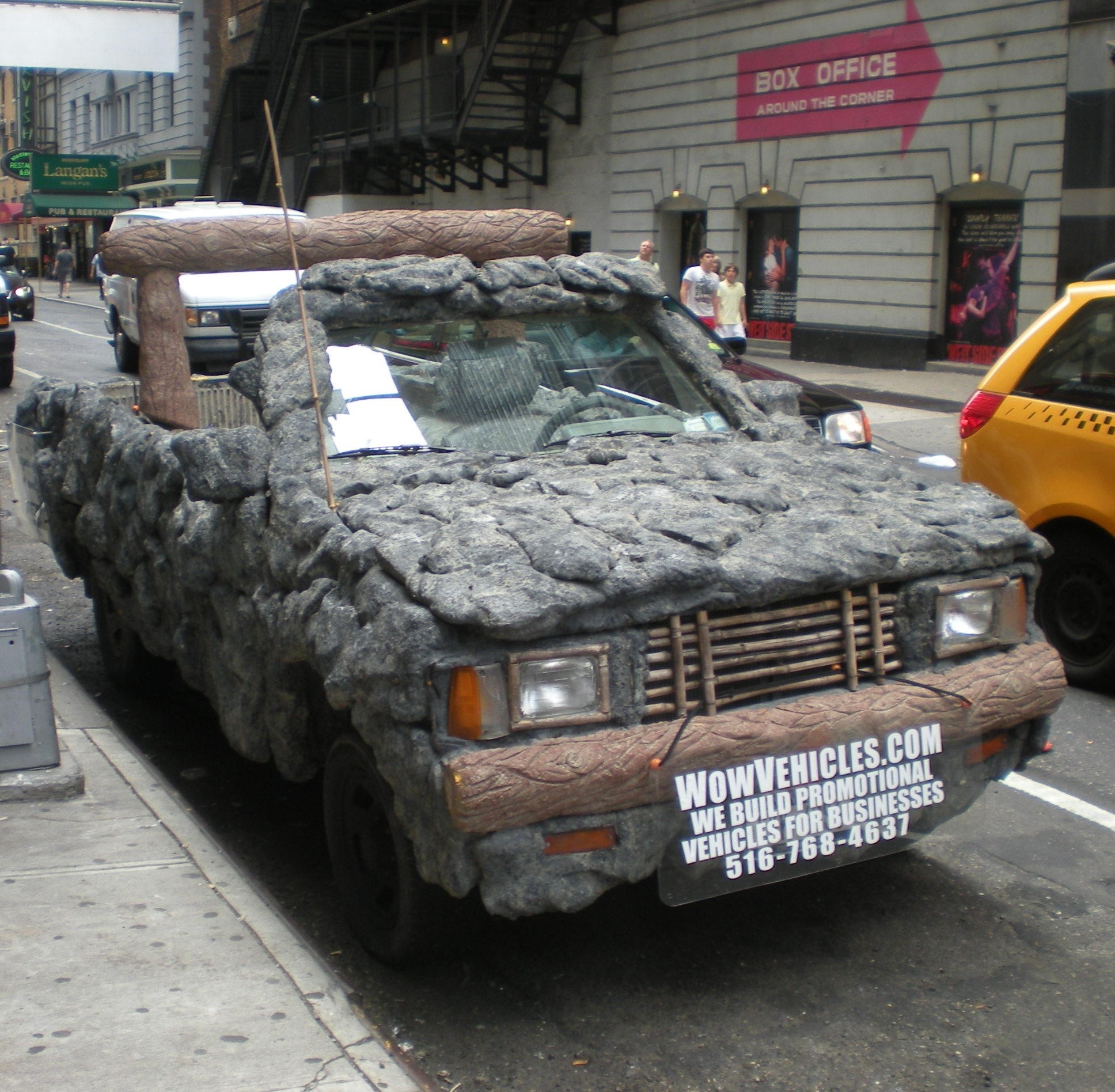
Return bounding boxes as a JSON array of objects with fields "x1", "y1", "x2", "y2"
[{"x1": 102, "y1": 194, "x2": 308, "y2": 370}]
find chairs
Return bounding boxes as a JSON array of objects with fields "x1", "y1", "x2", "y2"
[{"x1": 428, "y1": 348, "x2": 555, "y2": 460}]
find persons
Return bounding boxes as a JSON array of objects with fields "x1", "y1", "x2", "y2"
[
  {"x1": 629, "y1": 239, "x2": 660, "y2": 272},
  {"x1": 679, "y1": 248, "x2": 748, "y2": 340},
  {"x1": 90, "y1": 254, "x2": 105, "y2": 301},
  {"x1": 53, "y1": 242, "x2": 75, "y2": 298},
  {"x1": 958, "y1": 237, "x2": 1021, "y2": 344},
  {"x1": 759, "y1": 233, "x2": 796, "y2": 319}
]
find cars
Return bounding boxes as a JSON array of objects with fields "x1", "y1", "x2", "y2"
[
  {"x1": 958, "y1": 259, "x2": 1115, "y2": 691},
  {"x1": 0, "y1": 245, "x2": 34, "y2": 390}
]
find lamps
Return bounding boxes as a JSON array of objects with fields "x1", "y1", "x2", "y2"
[
  {"x1": 760, "y1": 178, "x2": 769, "y2": 195},
  {"x1": 672, "y1": 183, "x2": 682, "y2": 199},
  {"x1": 970, "y1": 163, "x2": 983, "y2": 184},
  {"x1": 565, "y1": 211, "x2": 572, "y2": 225}
]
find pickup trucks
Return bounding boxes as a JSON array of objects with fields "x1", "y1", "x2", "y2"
[{"x1": 9, "y1": 252, "x2": 1069, "y2": 963}]
